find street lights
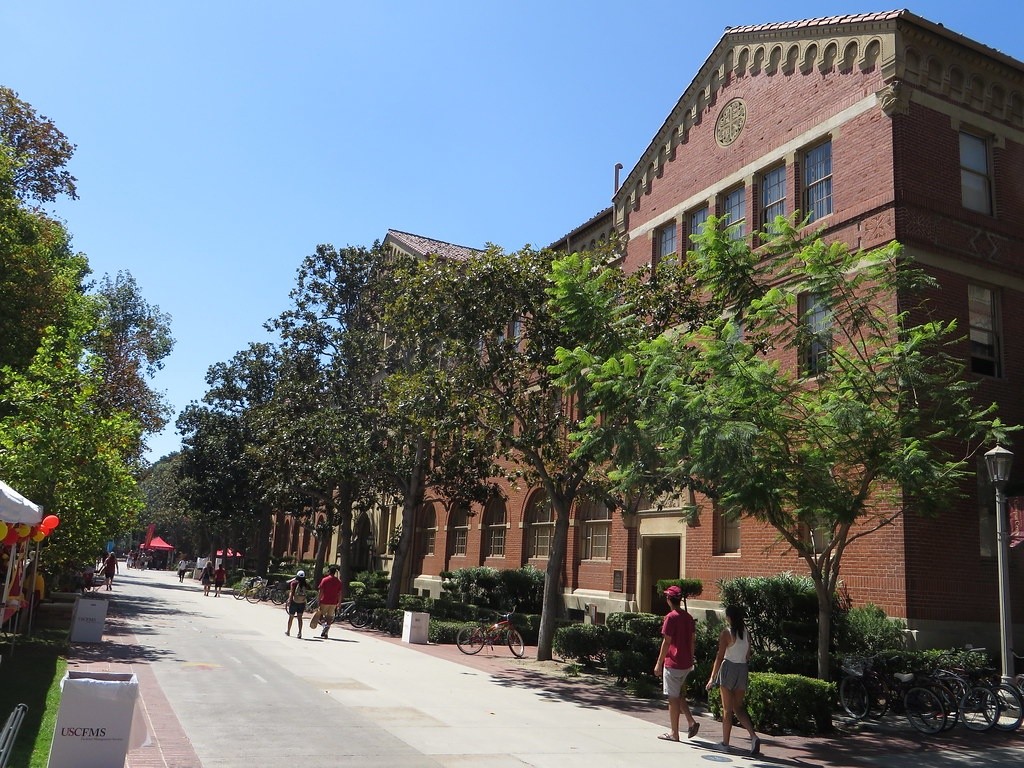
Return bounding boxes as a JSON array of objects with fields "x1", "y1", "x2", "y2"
[{"x1": 982, "y1": 436, "x2": 1024, "y2": 720}]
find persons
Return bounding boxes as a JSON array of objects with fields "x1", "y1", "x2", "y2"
[
  {"x1": 654, "y1": 586, "x2": 701, "y2": 742},
  {"x1": 704, "y1": 604, "x2": 761, "y2": 754},
  {"x1": 102, "y1": 549, "x2": 162, "y2": 590},
  {"x1": 198, "y1": 561, "x2": 226, "y2": 597},
  {"x1": 317, "y1": 566, "x2": 342, "y2": 638},
  {"x1": 284, "y1": 570, "x2": 307, "y2": 638},
  {"x1": 179, "y1": 558, "x2": 186, "y2": 583}
]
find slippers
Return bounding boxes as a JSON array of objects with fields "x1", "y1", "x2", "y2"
[
  {"x1": 688, "y1": 723, "x2": 700, "y2": 738},
  {"x1": 658, "y1": 733, "x2": 679, "y2": 741}
]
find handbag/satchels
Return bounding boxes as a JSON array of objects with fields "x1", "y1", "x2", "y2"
[
  {"x1": 209, "y1": 575, "x2": 215, "y2": 582},
  {"x1": 309, "y1": 607, "x2": 321, "y2": 629},
  {"x1": 98, "y1": 563, "x2": 108, "y2": 576}
]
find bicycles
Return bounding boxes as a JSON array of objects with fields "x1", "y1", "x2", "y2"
[
  {"x1": 456, "y1": 605, "x2": 524, "y2": 658},
  {"x1": 838, "y1": 646, "x2": 1024, "y2": 735},
  {"x1": 234, "y1": 576, "x2": 369, "y2": 628}
]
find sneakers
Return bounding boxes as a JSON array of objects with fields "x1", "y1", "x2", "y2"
[
  {"x1": 713, "y1": 742, "x2": 731, "y2": 753},
  {"x1": 751, "y1": 736, "x2": 761, "y2": 754}
]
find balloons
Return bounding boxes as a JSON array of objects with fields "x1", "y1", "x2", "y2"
[{"x1": 0, "y1": 516, "x2": 59, "y2": 546}]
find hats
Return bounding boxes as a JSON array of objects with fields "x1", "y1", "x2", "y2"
[
  {"x1": 664, "y1": 586, "x2": 682, "y2": 600},
  {"x1": 297, "y1": 571, "x2": 305, "y2": 577},
  {"x1": 110, "y1": 552, "x2": 115, "y2": 555}
]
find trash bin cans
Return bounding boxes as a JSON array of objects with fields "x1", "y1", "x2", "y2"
[
  {"x1": 193, "y1": 569, "x2": 201, "y2": 579},
  {"x1": 70, "y1": 596, "x2": 109, "y2": 642},
  {"x1": 401, "y1": 611, "x2": 430, "y2": 644},
  {"x1": 47, "y1": 671, "x2": 138, "y2": 768}
]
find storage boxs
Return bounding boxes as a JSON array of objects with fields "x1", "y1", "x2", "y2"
[
  {"x1": 70, "y1": 596, "x2": 109, "y2": 644},
  {"x1": 47, "y1": 670, "x2": 139, "y2": 768},
  {"x1": 402, "y1": 610, "x2": 430, "y2": 644}
]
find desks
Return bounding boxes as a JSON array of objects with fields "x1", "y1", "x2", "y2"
[{"x1": 0, "y1": 608, "x2": 19, "y2": 656}]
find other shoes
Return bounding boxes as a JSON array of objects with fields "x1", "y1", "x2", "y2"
[
  {"x1": 297, "y1": 633, "x2": 302, "y2": 639},
  {"x1": 214, "y1": 593, "x2": 220, "y2": 597},
  {"x1": 204, "y1": 593, "x2": 208, "y2": 596},
  {"x1": 179, "y1": 580, "x2": 183, "y2": 583},
  {"x1": 321, "y1": 627, "x2": 330, "y2": 638},
  {"x1": 285, "y1": 631, "x2": 290, "y2": 636},
  {"x1": 106, "y1": 585, "x2": 112, "y2": 591}
]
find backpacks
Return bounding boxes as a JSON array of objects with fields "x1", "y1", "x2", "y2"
[{"x1": 293, "y1": 580, "x2": 307, "y2": 603}]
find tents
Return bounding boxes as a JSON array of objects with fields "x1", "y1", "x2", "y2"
[
  {"x1": 0, "y1": 480, "x2": 42, "y2": 641},
  {"x1": 139, "y1": 537, "x2": 175, "y2": 571},
  {"x1": 210, "y1": 548, "x2": 242, "y2": 558}
]
